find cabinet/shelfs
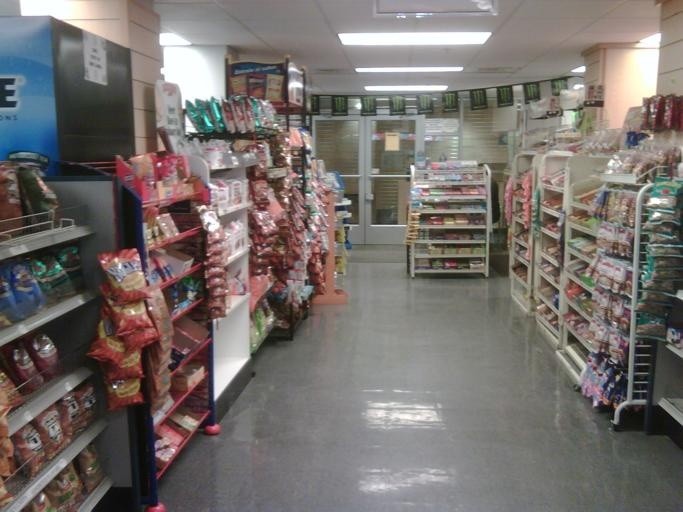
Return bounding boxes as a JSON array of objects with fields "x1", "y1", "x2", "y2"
[
  {"x1": 184, "y1": 131, "x2": 314, "y2": 363},
  {"x1": 507, "y1": 148, "x2": 579, "y2": 353},
  {"x1": 222, "y1": 56, "x2": 349, "y2": 304},
  {"x1": 403, "y1": 160, "x2": 492, "y2": 280},
  {"x1": 554, "y1": 147, "x2": 683, "y2": 438},
  {"x1": 0, "y1": 145, "x2": 257, "y2": 510}
]
